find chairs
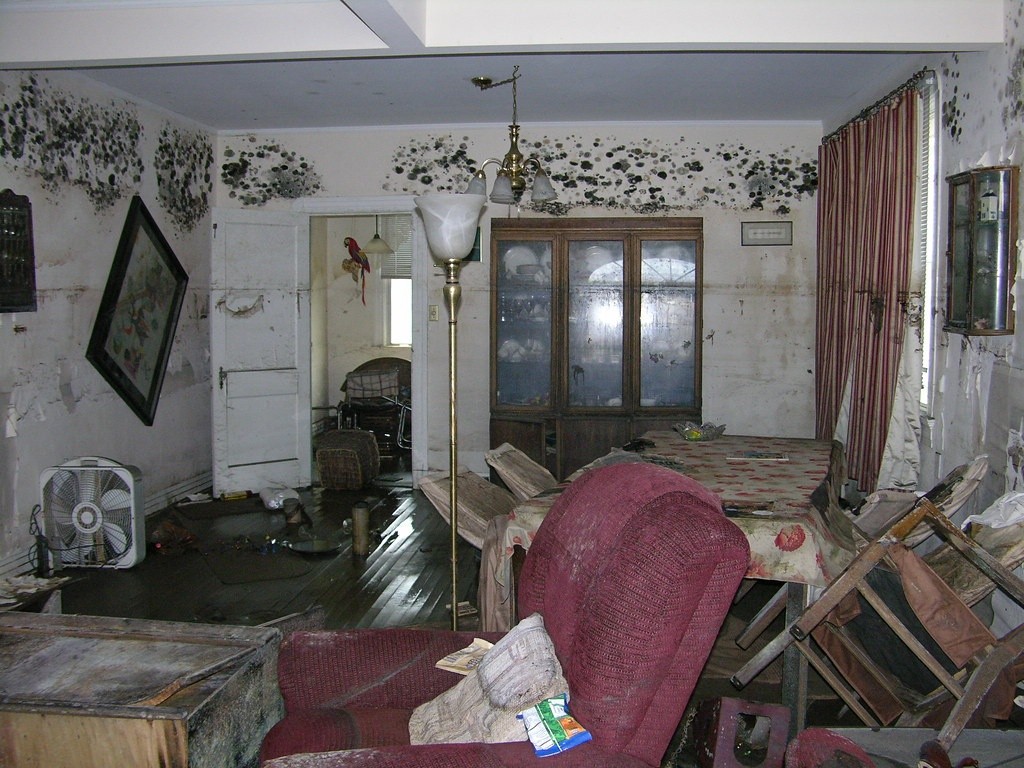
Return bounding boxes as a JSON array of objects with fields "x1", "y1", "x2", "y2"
[
  {"x1": 418, "y1": 469, "x2": 518, "y2": 549},
  {"x1": 877, "y1": 453, "x2": 988, "y2": 545},
  {"x1": 485, "y1": 442, "x2": 558, "y2": 500},
  {"x1": 923, "y1": 494, "x2": 1022, "y2": 605},
  {"x1": 735, "y1": 499, "x2": 1023, "y2": 767}
]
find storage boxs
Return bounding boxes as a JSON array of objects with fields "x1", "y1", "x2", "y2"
[{"x1": 317, "y1": 430, "x2": 380, "y2": 490}]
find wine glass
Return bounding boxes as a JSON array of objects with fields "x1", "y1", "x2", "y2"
[{"x1": 497, "y1": 291, "x2": 551, "y2": 321}]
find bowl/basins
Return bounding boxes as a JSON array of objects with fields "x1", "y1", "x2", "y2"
[{"x1": 496, "y1": 337, "x2": 682, "y2": 365}]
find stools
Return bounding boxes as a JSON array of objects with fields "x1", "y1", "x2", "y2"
[{"x1": 699, "y1": 694, "x2": 787, "y2": 768}]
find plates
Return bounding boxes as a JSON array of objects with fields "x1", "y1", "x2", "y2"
[
  {"x1": 500, "y1": 244, "x2": 693, "y2": 280},
  {"x1": 287, "y1": 539, "x2": 342, "y2": 555}
]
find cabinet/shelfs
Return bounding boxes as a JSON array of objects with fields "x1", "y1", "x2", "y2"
[
  {"x1": 942, "y1": 167, "x2": 1020, "y2": 338},
  {"x1": 0, "y1": 612, "x2": 278, "y2": 768},
  {"x1": 491, "y1": 218, "x2": 703, "y2": 490}
]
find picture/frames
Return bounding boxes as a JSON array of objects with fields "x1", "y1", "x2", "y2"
[{"x1": 87, "y1": 197, "x2": 190, "y2": 424}]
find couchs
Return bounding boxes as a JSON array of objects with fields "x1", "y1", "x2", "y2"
[{"x1": 262, "y1": 467, "x2": 749, "y2": 768}]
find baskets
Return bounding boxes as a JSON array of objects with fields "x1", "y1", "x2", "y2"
[{"x1": 316, "y1": 429, "x2": 382, "y2": 491}]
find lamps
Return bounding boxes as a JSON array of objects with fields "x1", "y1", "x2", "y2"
[
  {"x1": 415, "y1": 194, "x2": 490, "y2": 631},
  {"x1": 465, "y1": 65, "x2": 556, "y2": 206},
  {"x1": 359, "y1": 216, "x2": 392, "y2": 255}
]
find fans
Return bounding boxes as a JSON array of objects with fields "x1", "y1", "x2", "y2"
[{"x1": 43, "y1": 458, "x2": 144, "y2": 569}]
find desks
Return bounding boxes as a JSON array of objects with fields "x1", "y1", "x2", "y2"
[
  {"x1": 481, "y1": 430, "x2": 856, "y2": 742},
  {"x1": 802, "y1": 726, "x2": 1024, "y2": 768}
]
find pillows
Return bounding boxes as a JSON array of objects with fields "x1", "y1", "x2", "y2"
[{"x1": 409, "y1": 613, "x2": 577, "y2": 741}]
